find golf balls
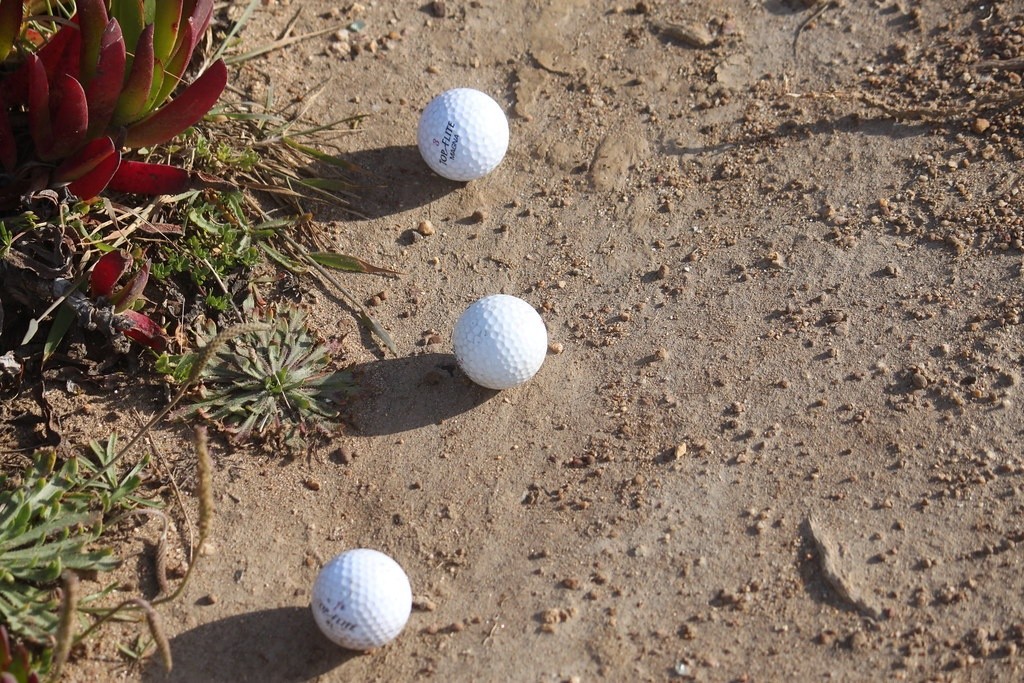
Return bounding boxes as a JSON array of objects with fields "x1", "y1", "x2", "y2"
[
  {"x1": 416, "y1": 87, "x2": 511, "y2": 180},
  {"x1": 310, "y1": 549, "x2": 414, "y2": 649},
  {"x1": 452, "y1": 294, "x2": 549, "y2": 389}
]
